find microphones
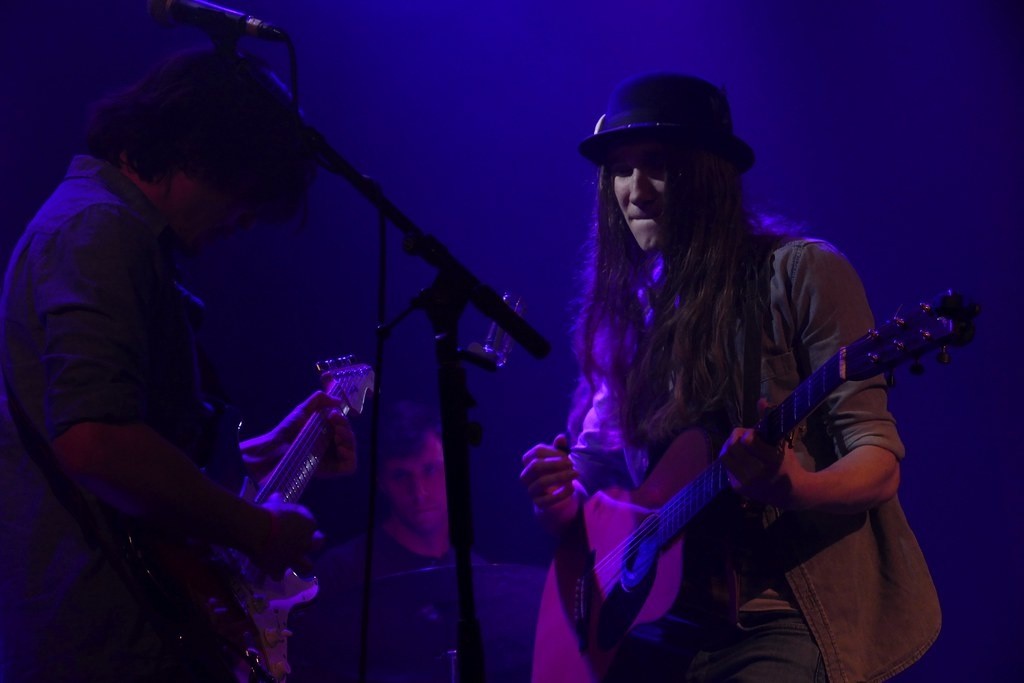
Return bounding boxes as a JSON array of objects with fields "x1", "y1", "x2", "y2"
[{"x1": 147, "y1": 0, "x2": 283, "y2": 42}]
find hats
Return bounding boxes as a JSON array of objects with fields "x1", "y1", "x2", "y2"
[{"x1": 579, "y1": 66, "x2": 756, "y2": 174}]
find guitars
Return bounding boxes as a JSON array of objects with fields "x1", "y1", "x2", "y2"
[
  {"x1": 205, "y1": 352, "x2": 377, "y2": 683},
  {"x1": 525, "y1": 288, "x2": 978, "y2": 683}
]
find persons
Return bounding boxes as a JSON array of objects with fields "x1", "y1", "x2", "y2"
[
  {"x1": 518, "y1": 76, "x2": 942, "y2": 683},
  {"x1": 0, "y1": 48, "x2": 356, "y2": 683},
  {"x1": 316, "y1": 409, "x2": 527, "y2": 683}
]
